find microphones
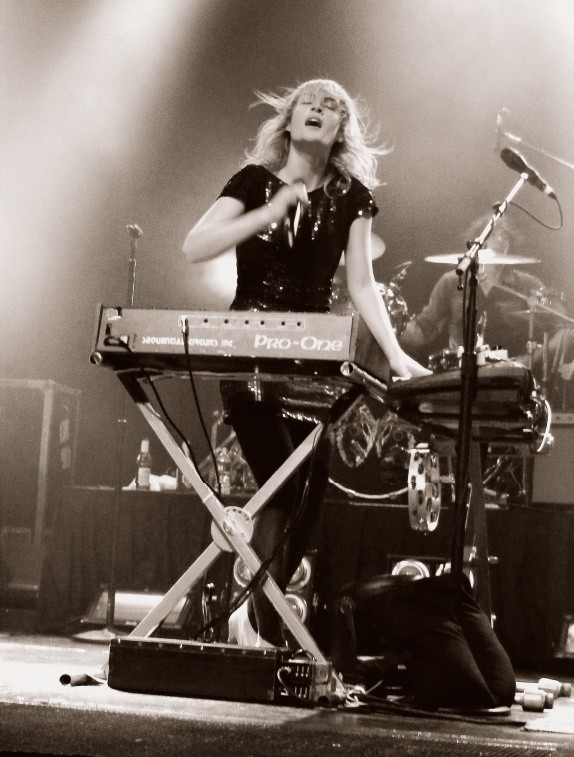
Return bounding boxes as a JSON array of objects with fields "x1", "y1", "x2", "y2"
[
  {"x1": 284, "y1": 178, "x2": 308, "y2": 249},
  {"x1": 499, "y1": 147, "x2": 557, "y2": 199},
  {"x1": 125, "y1": 224, "x2": 144, "y2": 239}
]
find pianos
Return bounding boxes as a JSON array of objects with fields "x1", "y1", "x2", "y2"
[
  {"x1": 392, "y1": 357, "x2": 554, "y2": 708},
  {"x1": 87, "y1": 300, "x2": 389, "y2": 711}
]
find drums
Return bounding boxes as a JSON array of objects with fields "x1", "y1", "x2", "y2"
[{"x1": 429, "y1": 345, "x2": 512, "y2": 374}]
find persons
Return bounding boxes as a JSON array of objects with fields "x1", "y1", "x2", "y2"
[
  {"x1": 183, "y1": 80, "x2": 433, "y2": 649},
  {"x1": 398, "y1": 215, "x2": 564, "y2": 382}
]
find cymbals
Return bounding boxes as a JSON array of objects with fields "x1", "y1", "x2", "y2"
[
  {"x1": 422, "y1": 249, "x2": 543, "y2": 266},
  {"x1": 489, "y1": 281, "x2": 574, "y2": 325}
]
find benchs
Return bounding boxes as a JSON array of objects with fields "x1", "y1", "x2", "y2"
[{"x1": 36, "y1": 485, "x2": 574, "y2": 670}]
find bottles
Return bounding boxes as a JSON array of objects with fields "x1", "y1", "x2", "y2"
[
  {"x1": 216, "y1": 447, "x2": 231, "y2": 495},
  {"x1": 136, "y1": 438, "x2": 151, "y2": 490},
  {"x1": 176, "y1": 441, "x2": 192, "y2": 493}
]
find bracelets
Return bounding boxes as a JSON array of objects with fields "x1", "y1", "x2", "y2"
[{"x1": 264, "y1": 201, "x2": 278, "y2": 232}]
